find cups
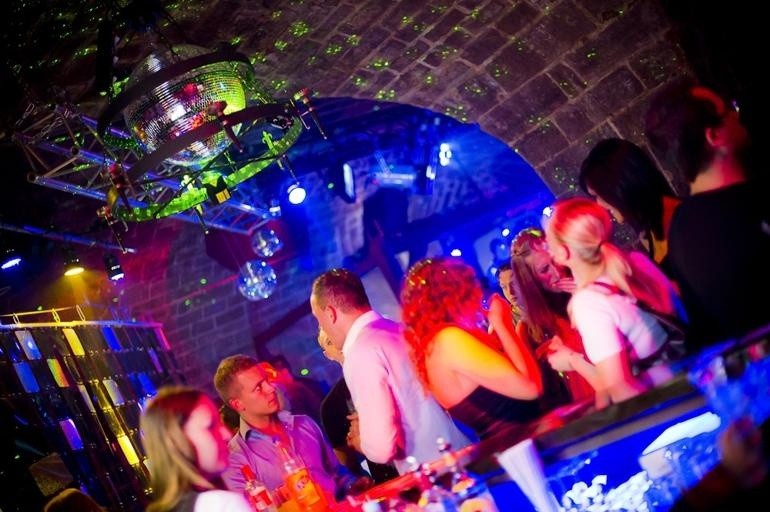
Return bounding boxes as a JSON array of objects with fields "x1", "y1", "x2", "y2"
[{"x1": 497, "y1": 438, "x2": 562, "y2": 512}]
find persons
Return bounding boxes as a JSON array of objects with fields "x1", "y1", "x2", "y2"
[{"x1": 140, "y1": 76, "x2": 770, "y2": 512}]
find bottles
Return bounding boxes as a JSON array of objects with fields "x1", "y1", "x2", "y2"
[{"x1": 241, "y1": 434, "x2": 499, "y2": 512}]
